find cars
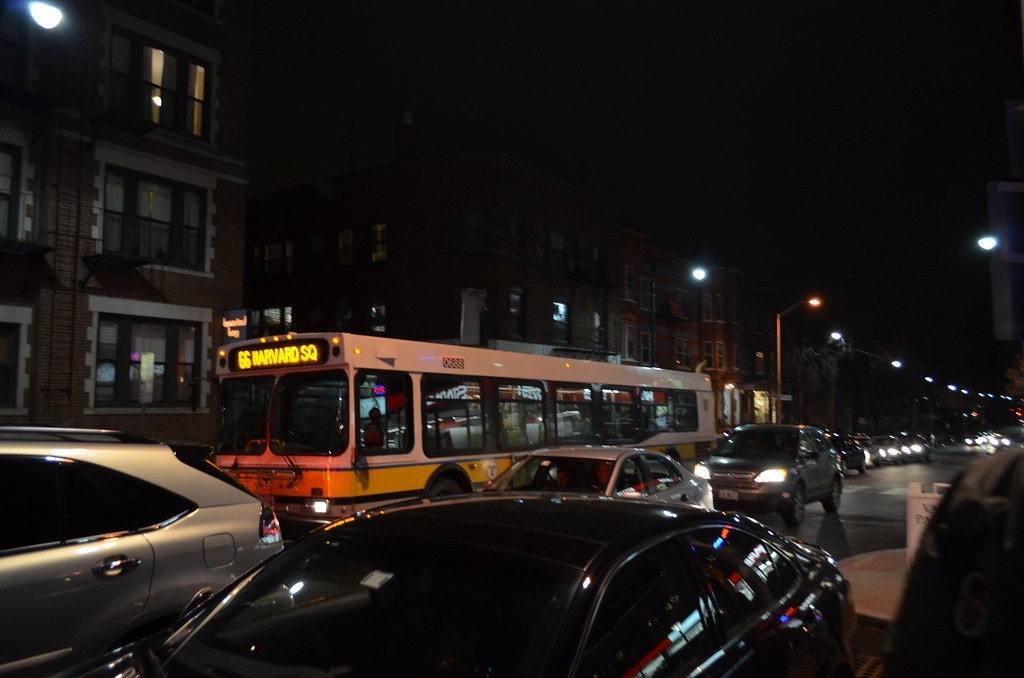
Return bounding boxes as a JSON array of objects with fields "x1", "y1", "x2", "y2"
[
  {"x1": 479, "y1": 446, "x2": 714, "y2": 512},
  {"x1": 829, "y1": 436, "x2": 867, "y2": 474},
  {"x1": 853, "y1": 436, "x2": 931, "y2": 465},
  {"x1": 50, "y1": 494, "x2": 857, "y2": 678},
  {"x1": 966, "y1": 432, "x2": 1008, "y2": 449}
]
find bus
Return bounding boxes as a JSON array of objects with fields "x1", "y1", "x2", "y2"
[{"x1": 189, "y1": 332, "x2": 717, "y2": 526}]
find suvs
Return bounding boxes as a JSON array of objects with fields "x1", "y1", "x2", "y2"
[
  {"x1": 0, "y1": 424, "x2": 286, "y2": 677},
  {"x1": 693, "y1": 422, "x2": 845, "y2": 525}
]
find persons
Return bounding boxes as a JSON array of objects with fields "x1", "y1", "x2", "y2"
[
  {"x1": 543, "y1": 467, "x2": 576, "y2": 492},
  {"x1": 585, "y1": 464, "x2": 630, "y2": 496}
]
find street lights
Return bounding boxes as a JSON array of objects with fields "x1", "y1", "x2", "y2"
[
  {"x1": 777, "y1": 297, "x2": 822, "y2": 423},
  {"x1": 650, "y1": 267, "x2": 705, "y2": 364}
]
